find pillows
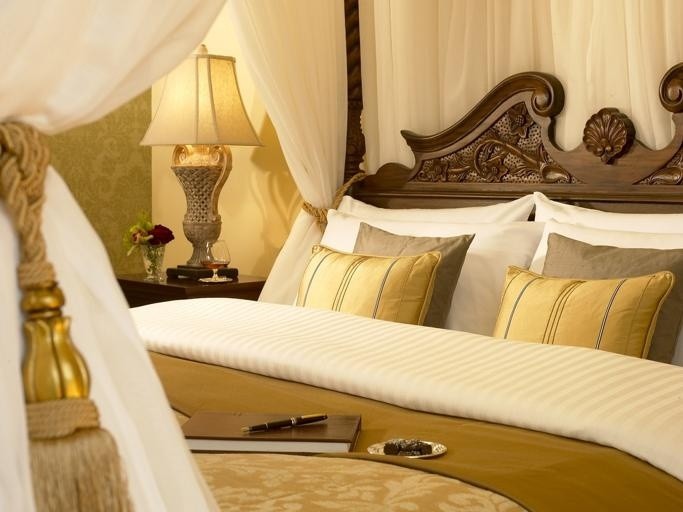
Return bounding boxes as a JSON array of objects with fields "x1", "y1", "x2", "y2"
[
  {"x1": 541, "y1": 233, "x2": 681, "y2": 365},
  {"x1": 319, "y1": 209, "x2": 545, "y2": 338},
  {"x1": 533, "y1": 191, "x2": 681, "y2": 233},
  {"x1": 337, "y1": 194, "x2": 535, "y2": 222},
  {"x1": 529, "y1": 218, "x2": 681, "y2": 275},
  {"x1": 352, "y1": 222, "x2": 477, "y2": 329},
  {"x1": 295, "y1": 243, "x2": 442, "y2": 326},
  {"x1": 491, "y1": 265, "x2": 675, "y2": 359}
]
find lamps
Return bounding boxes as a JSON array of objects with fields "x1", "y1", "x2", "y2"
[{"x1": 137, "y1": 43, "x2": 266, "y2": 280}]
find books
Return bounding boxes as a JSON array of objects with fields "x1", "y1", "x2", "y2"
[{"x1": 180, "y1": 409, "x2": 362, "y2": 452}]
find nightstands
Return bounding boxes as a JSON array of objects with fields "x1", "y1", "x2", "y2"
[{"x1": 114, "y1": 273, "x2": 268, "y2": 309}]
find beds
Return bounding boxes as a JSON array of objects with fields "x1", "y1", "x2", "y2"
[{"x1": 101, "y1": 69, "x2": 682, "y2": 511}]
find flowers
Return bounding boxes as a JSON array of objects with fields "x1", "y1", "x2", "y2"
[{"x1": 121, "y1": 207, "x2": 175, "y2": 272}]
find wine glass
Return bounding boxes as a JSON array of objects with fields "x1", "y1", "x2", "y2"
[{"x1": 204, "y1": 239, "x2": 232, "y2": 282}]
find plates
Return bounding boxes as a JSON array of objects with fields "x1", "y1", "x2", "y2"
[{"x1": 366, "y1": 438, "x2": 449, "y2": 459}]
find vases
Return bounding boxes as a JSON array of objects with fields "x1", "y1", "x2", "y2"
[{"x1": 139, "y1": 245, "x2": 166, "y2": 279}]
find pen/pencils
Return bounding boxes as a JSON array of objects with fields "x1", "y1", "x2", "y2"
[{"x1": 241, "y1": 413, "x2": 328, "y2": 432}]
